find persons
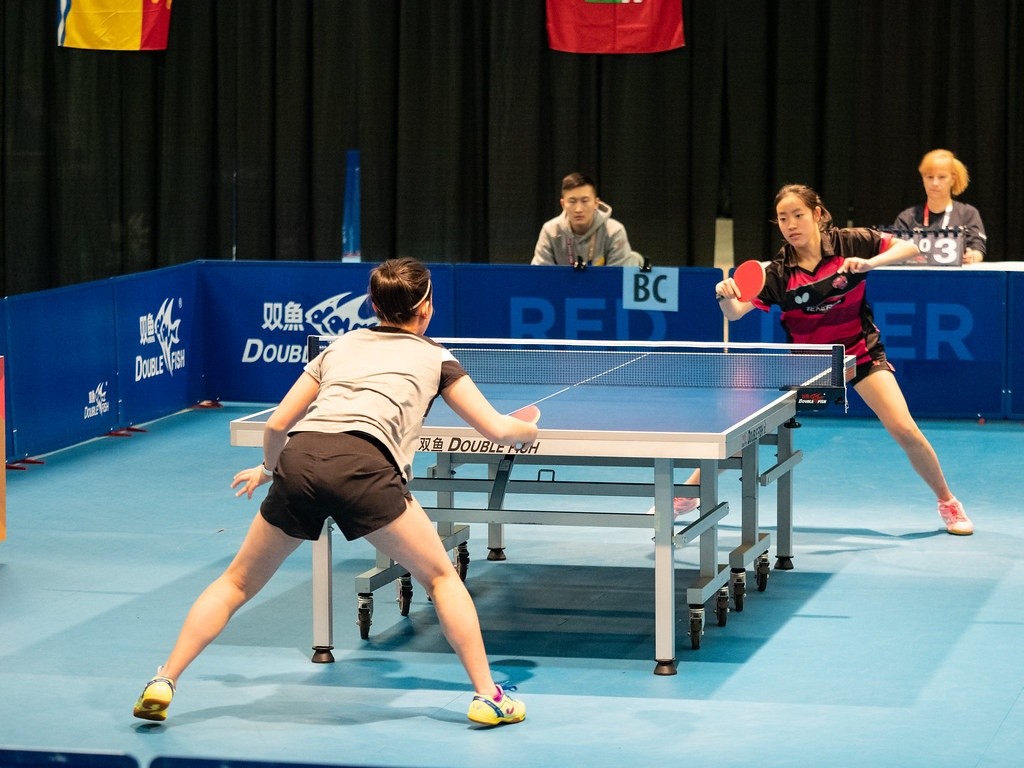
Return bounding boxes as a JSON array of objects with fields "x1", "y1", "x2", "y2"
[
  {"x1": 893, "y1": 148, "x2": 988, "y2": 265},
  {"x1": 647, "y1": 183, "x2": 976, "y2": 537},
  {"x1": 134, "y1": 256, "x2": 539, "y2": 724},
  {"x1": 531, "y1": 172, "x2": 646, "y2": 266}
]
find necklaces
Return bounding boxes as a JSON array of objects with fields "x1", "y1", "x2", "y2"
[{"x1": 924, "y1": 198, "x2": 953, "y2": 229}]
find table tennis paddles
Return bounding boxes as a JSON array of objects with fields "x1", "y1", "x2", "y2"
[
  {"x1": 508, "y1": 404, "x2": 541, "y2": 451},
  {"x1": 715, "y1": 259, "x2": 767, "y2": 303}
]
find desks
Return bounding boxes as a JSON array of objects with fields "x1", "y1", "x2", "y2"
[
  {"x1": 874, "y1": 261, "x2": 1024, "y2": 271},
  {"x1": 228, "y1": 348, "x2": 856, "y2": 677}
]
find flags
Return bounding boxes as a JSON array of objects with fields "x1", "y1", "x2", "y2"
[
  {"x1": 549, "y1": 0, "x2": 690, "y2": 54},
  {"x1": 56, "y1": 0, "x2": 171, "y2": 52}
]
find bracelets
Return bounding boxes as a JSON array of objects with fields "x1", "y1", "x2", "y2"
[{"x1": 261, "y1": 462, "x2": 275, "y2": 476}]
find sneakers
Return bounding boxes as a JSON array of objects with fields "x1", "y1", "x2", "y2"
[
  {"x1": 647, "y1": 497, "x2": 700, "y2": 519},
  {"x1": 133, "y1": 665, "x2": 176, "y2": 721},
  {"x1": 937, "y1": 496, "x2": 974, "y2": 534},
  {"x1": 467, "y1": 684, "x2": 526, "y2": 725}
]
